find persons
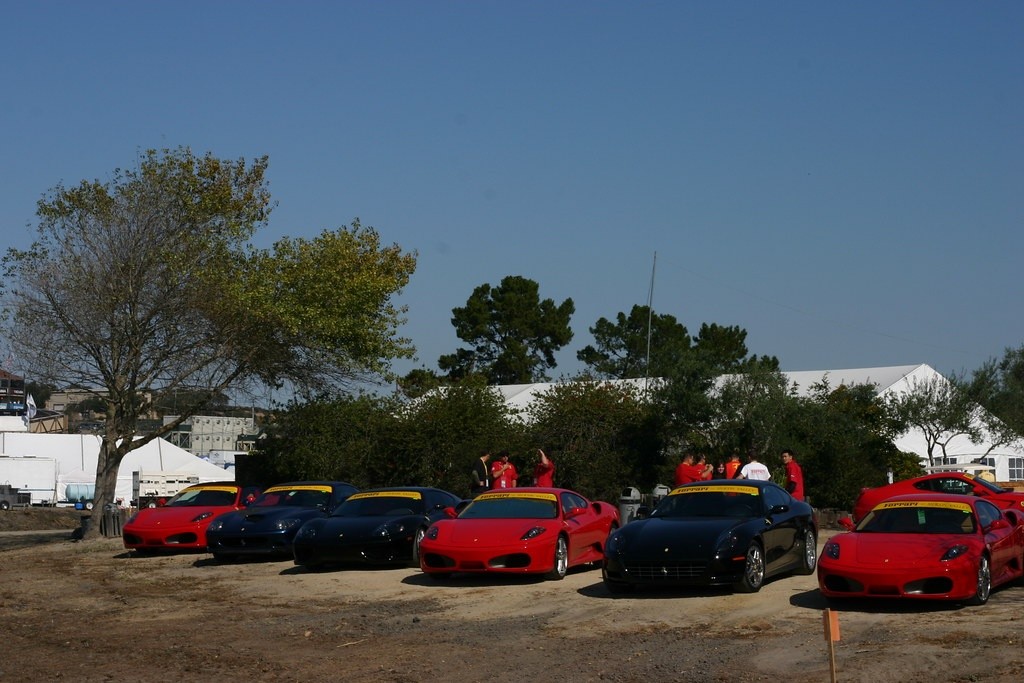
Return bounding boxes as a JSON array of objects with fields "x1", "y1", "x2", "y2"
[
  {"x1": 713, "y1": 461, "x2": 726, "y2": 479},
  {"x1": 978, "y1": 470, "x2": 994, "y2": 483},
  {"x1": 534, "y1": 448, "x2": 554, "y2": 488},
  {"x1": 735, "y1": 451, "x2": 771, "y2": 482},
  {"x1": 693, "y1": 453, "x2": 713, "y2": 481},
  {"x1": 675, "y1": 453, "x2": 701, "y2": 487},
  {"x1": 781, "y1": 449, "x2": 804, "y2": 502},
  {"x1": 491, "y1": 450, "x2": 518, "y2": 489},
  {"x1": 472, "y1": 450, "x2": 493, "y2": 496},
  {"x1": 725, "y1": 452, "x2": 742, "y2": 479}
]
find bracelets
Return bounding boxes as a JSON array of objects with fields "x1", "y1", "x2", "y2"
[{"x1": 709, "y1": 468, "x2": 712, "y2": 471}]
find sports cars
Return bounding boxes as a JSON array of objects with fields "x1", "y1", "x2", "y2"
[
  {"x1": 205, "y1": 481, "x2": 364, "y2": 560},
  {"x1": 817, "y1": 493, "x2": 1024, "y2": 607},
  {"x1": 603, "y1": 478, "x2": 820, "y2": 592},
  {"x1": 852, "y1": 470, "x2": 1024, "y2": 526},
  {"x1": 121, "y1": 481, "x2": 299, "y2": 552},
  {"x1": 292, "y1": 486, "x2": 472, "y2": 568},
  {"x1": 418, "y1": 486, "x2": 621, "y2": 579}
]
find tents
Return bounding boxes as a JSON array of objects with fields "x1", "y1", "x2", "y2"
[{"x1": 923, "y1": 463, "x2": 996, "y2": 485}]
